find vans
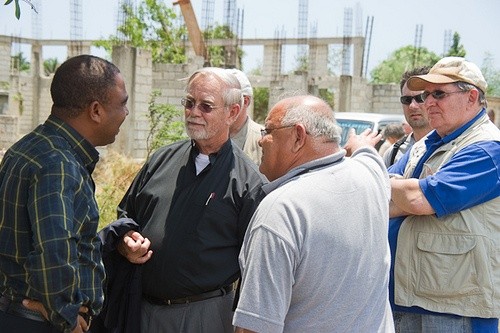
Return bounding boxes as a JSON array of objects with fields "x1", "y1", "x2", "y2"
[{"x1": 334, "y1": 111, "x2": 407, "y2": 151}]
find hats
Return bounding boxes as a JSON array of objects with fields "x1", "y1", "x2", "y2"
[{"x1": 407, "y1": 56, "x2": 488, "y2": 94}]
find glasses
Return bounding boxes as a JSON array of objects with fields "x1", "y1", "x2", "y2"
[
  {"x1": 400, "y1": 94, "x2": 425, "y2": 103},
  {"x1": 260, "y1": 124, "x2": 296, "y2": 139},
  {"x1": 181, "y1": 97, "x2": 221, "y2": 113},
  {"x1": 421, "y1": 90, "x2": 468, "y2": 102}
]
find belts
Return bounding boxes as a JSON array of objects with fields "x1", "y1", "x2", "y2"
[
  {"x1": 0, "y1": 301, "x2": 93, "y2": 324},
  {"x1": 141, "y1": 284, "x2": 233, "y2": 306}
]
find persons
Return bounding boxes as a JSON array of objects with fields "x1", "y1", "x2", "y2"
[
  {"x1": 386, "y1": 56, "x2": 500, "y2": 333},
  {"x1": 222, "y1": 68, "x2": 276, "y2": 187},
  {"x1": 0, "y1": 54, "x2": 128, "y2": 333},
  {"x1": 114, "y1": 68, "x2": 272, "y2": 333},
  {"x1": 363, "y1": 121, "x2": 413, "y2": 172},
  {"x1": 380, "y1": 65, "x2": 434, "y2": 169},
  {"x1": 231, "y1": 92, "x2": 400, "y2": 332}
]
